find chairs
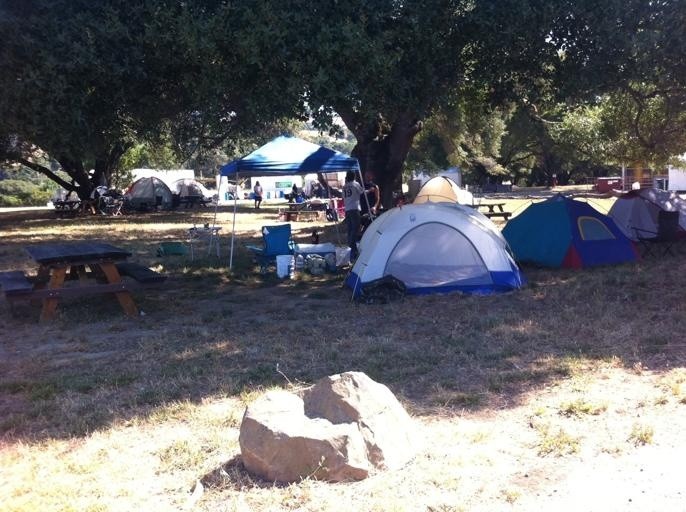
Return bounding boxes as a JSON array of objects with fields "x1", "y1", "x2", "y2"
[
  {"x1": 245, "y1": 224, "x2": 295, "y2": 277},
  {"x1": 630, "y1": 210, "x2": 686, "y2": 262}
]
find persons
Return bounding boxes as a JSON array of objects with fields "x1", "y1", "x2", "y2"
[
  {"x1": 290, "y1": 172, "x2": 382, "y2": 259},
  {"x1": 88, "y1": 186, "x2": 129, "y2": 215},
  {"x1": 253, "y1": 181, "x2": 263, "y2": 209}
]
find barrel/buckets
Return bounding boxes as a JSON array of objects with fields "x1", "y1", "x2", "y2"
[{"x1": 277, "y1": 255, "x2": 295, "y2": 279}]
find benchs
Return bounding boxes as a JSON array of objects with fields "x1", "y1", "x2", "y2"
[
  {"x1": 285, "y1": 211, "x2": 319, "y2": 221},
  {"x1": 0, "y1": 269, "x2": 33, "y2": 304},
  {"x1": 116, "y1": 261, "x2": 167, "y2": 294},
  {"x1": 482, "y1": 212, "x2": 512, "y2": 221}
]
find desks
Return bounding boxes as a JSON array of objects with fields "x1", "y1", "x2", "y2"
[
  {"x1": 20, "y1": 240, "x2": 139, "y2": 325},
  {"x1": 473, "y1": 203, "x2": 507, "y2": 212},
  {"x1": 285, "y1": 202, "x2": 306, "y2": 211}
]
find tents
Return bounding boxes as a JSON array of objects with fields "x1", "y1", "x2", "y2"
[
  {"x1": 500, "y1": 191, "x2": 645, "y2": 272},
  {"x1": 169, "y1": 178, "x2": 217, "y2": 208},
  {"x1": 345, "y1": 204, "x2": 528, "y2": 301},
  {"x1": 413, "y1": 175, "x2": 474, "y2": 204},
  {"x1": 608, "y1": 188, "x2": 686, "y2": 244},
  {"x1": 357, "y1": 200, "x2": 505, "y2": 243},
  {"x1": 124, "y1": 176, "x2": 173, "y2": 211}
]
